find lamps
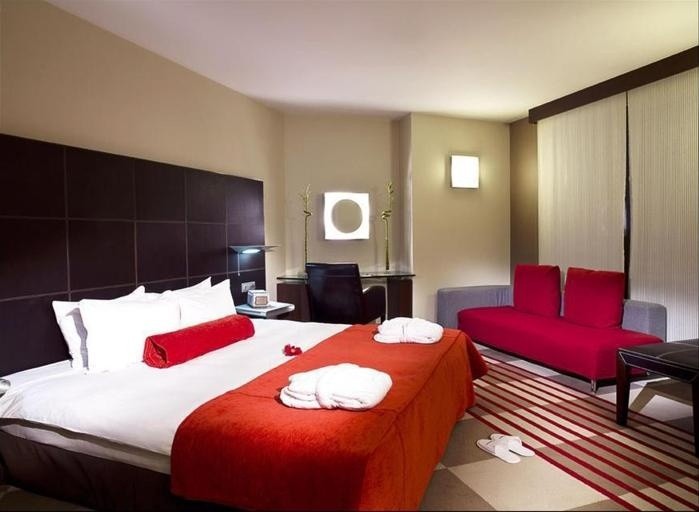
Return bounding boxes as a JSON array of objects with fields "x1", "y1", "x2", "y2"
[{"x1": 229, "y1": 244, "x2": 274, "y2": 255}]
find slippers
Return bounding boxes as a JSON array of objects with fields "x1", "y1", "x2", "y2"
[
  {"x1": 475, "y1": 438, "x2": 521, "y2": 464},
  {"x1": 488, "y1": 433, "x2": 535, "y2": 458}
]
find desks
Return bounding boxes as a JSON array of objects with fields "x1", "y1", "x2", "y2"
[
  {"x1": 613, "y1": 337, "x2": 699, "y2": 461},
  {"x1": 274, "y1": 271, "x2": 417, "y2": 326}
]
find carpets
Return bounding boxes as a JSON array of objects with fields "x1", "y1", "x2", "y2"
[{"x1": 461, "y1": 352, "x2": 699, "y2": 512}]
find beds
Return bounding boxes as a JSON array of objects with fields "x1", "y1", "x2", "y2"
[{"x1": 0, "y1": 316, "x2": 490, "y2": 510}]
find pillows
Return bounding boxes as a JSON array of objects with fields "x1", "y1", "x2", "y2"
[
  {"x1": 75, "y1": 291, "x2": 178, "y2": 372},
  {"x1": 512, "y1": 260, "x2": 564, "y2": 316},
  {"x1": 49, "y1": 285, "x2": 147, "y2": 373},
  {"x1": 137, "y1": 311, "x2": 259, "y2": 367},
  {"x1": 561, "y1": 267, "x2": 630, "y2": 331},
  {"x1": 172, "y1": 275, "x2": 213, "y2": 295},
  {"x1": 164, "y1": 279, "x2": 236, "y2": 329}
]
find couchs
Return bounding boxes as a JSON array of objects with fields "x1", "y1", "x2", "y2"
[{"x1": 438, "y1": 280, "x2": 670, "y2": 398}]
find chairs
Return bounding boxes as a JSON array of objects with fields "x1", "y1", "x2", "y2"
[{"x1": 304, "y1": 261, "x2": 387, "y2": 325}]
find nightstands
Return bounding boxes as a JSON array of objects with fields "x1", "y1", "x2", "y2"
[{"x1": 236, "y1": 302, "x2": 296, "y2": 320}]
